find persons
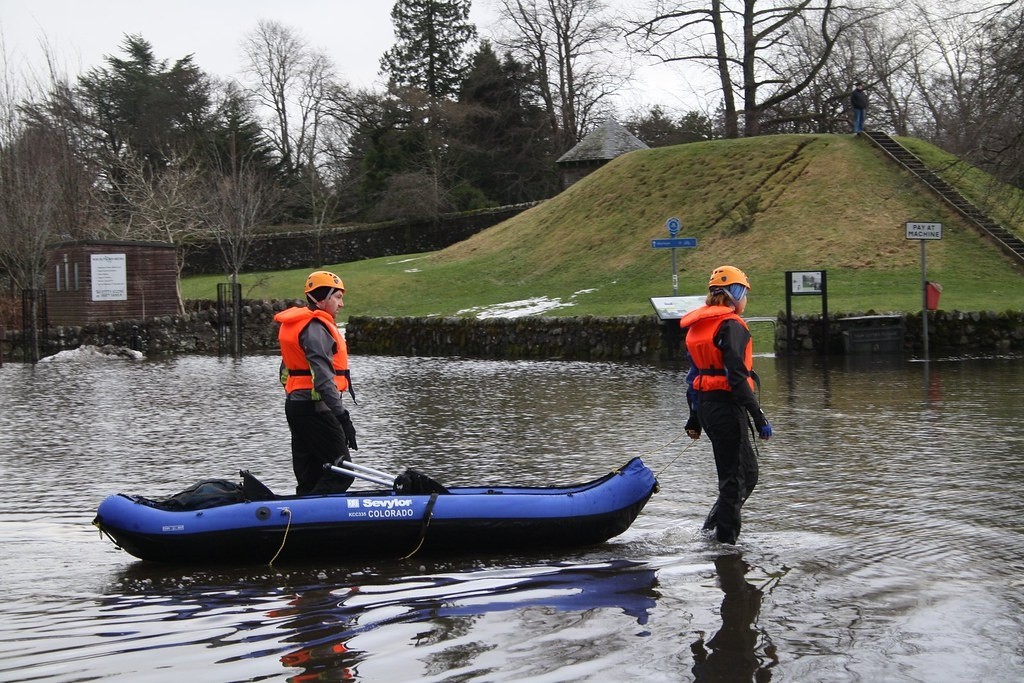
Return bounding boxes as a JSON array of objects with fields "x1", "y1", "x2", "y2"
[
  {"x1": 680, "y1": 266, "x2": 773, "y2": 545},
  {"x1": 274, "y1": 271, "x2": 357, "y2": 496}
]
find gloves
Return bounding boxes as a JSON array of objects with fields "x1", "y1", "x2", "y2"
[{"x1": 337, "y1": 408, "x2": 357, "y2": 450}]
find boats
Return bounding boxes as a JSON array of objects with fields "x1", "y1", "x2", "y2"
[{"x1": 90, "y1": 457, "x2": 657, "y2": 569}]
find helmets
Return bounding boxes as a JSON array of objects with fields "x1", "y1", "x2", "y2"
[
  {"x1": 709, "y1": 265, "x2": 752, "y2": 291},
  {"x1": 305, "y1": 270, "x2": 345, "y2": 294}
]
